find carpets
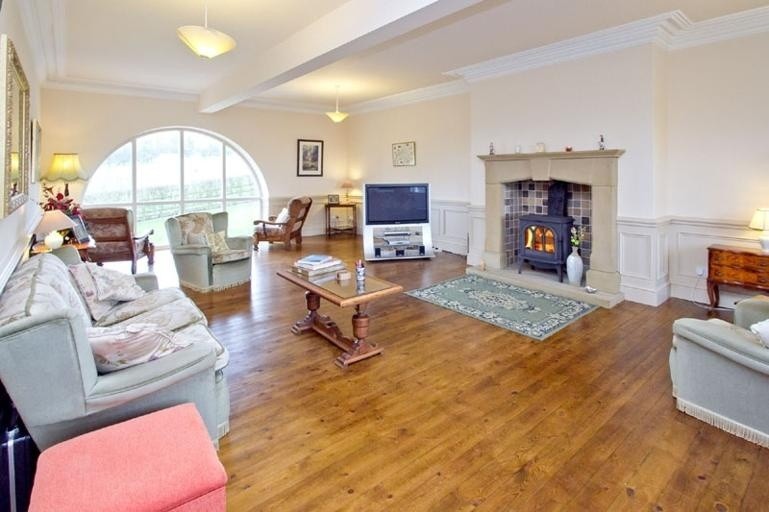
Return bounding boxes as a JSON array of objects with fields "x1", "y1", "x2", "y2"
[{"x1": 403, "y1": 274, "x2": 603, "y2": 341}]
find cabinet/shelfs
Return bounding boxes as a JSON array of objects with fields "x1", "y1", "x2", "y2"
[
  {"x1": 30, "y1": 235, "x2": 96, "y2": 263},
  {"x1": 516, "y1": 214, "x2": 575, "y2": 284},
  {"x1": 362, "y1": 222, "x2": 437, "y2": 262}
]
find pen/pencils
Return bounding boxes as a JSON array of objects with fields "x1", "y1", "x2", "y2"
[{"x1": 355, "y1": 259, "x2": 364, "y2": 268}]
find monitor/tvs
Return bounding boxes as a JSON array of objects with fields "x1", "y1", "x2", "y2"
[{"x1": 364, "y1": 183, "x2": 431, "y2": 227}]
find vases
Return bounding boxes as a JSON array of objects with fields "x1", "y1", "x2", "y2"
[
  {"x1": 58, "y1": 229, "x2": 75, "y2": 246},
  {"x1": 565, "y1": 245, "x2": 584, "y2": 287}
]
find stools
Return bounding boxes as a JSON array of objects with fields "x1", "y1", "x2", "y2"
[{"x1": 25, "y1": 402, "x2": 228, "y2": 511}]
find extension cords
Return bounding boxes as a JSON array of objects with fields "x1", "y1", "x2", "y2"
[{"x1": 433, "y1": 247, "x2": 442, "y2": 253}]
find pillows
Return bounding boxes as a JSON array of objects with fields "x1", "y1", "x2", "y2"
[
  {"x1": 187, "y1": 231, "x2": 209, "y2": 244},
  {"x1": 85, "y1": 261, "x2": 149, "y2": 303},
  {"x1": 85, "y1": 323, "x2": 194, "y2": 374},
  {"x1": 274, "y1": 207, "x2": 290, "y2": 227},
  {"x1": 67, "y1": 260, "x2": 119, "y2": 322},
  {"x1": 208, "y1": 229, "x2": 229, "y2": 252},
  {"x1": 749, "y1": 318, "x2": 769, "y2": 350}
]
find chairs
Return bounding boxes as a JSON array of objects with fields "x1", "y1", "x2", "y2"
[{"x1": 252, "y1": 195, "x2": 312, "y2": 251}]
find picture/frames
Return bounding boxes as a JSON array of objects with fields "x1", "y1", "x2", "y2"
[
  {"x1": 30, "y1": 117, "x2": 43, "y2": 184},
  {"x1": 68, "y1": 214, "x2": 91, "y2": 243},
  {"x1": 296, "y1": 138, "x2": 324, "y2": 178},
  {"x1": 391, "y1": 141, "x2": 416, "y2": 167},
  {"x1": 1, "y1": 33, "x2": 31, "y2": 216},
  {"x1": 328, "y1": 194, "x2": 339, "y2": 204}
]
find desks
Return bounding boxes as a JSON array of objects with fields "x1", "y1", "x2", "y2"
[
  {"x1": 325, "y1": 203, "x2": 358, "y2": 240},
  {"x1": 705, "y1": 245, "x2": 768, "y2": 319}
]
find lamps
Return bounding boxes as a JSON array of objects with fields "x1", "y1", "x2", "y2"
[
  {"x1": 748, "y1": 209, "x2": 769, "y2": 250},
  {"x1": 31, "y1": 209, "x2": 78, "y2": 250},
  {"x1": 324, "y1": 85, "x2": 350, "y2": 124},
  {"x1": 10, "y1": 152, "x2": 19, "y2": 197},
  {"x1": 39, "y1": 153, "x2": 91, "y2": 198},
  {"x1": 176, "y1": 0, "x2": 237, "y2": 62},
  {"x1": 340, "y1": 179, "x2": 354, "y2": 202}
]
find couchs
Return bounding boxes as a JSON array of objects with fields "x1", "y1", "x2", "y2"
[
  {"x1": 79, "y1": 207, "x2": 156, "y2": 275},
  {"x1": 0, "y1": 244, "x2": 230, "y2": 457},
  {"x1": 164, "y1": 211, "x2": 254, "y2": 294},
  {"x1": 668, "y1": 294, "x2": 769, "y2": 451}
]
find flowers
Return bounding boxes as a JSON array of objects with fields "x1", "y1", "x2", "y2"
[
  {"x1": 568, "y1": 224, "x2": 588, "y2": 249},
  {"x1": 37, "y1": 184, "x2": 81, "y2": 215}
]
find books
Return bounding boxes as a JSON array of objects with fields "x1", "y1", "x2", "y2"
[{"x1": 292, "y1": 255, "x2": 345, "y2": 277}]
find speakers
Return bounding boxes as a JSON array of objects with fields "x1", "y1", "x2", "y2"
[
  {"x1": 375, "y1": 248, "x2": 381, "y2": 258},
  {"x1": 419, "y1": 246, "x2": 425, "y2": 256},
  {"x1": 396, "y1": 250, "x2": 405, "y2": 256}
]
